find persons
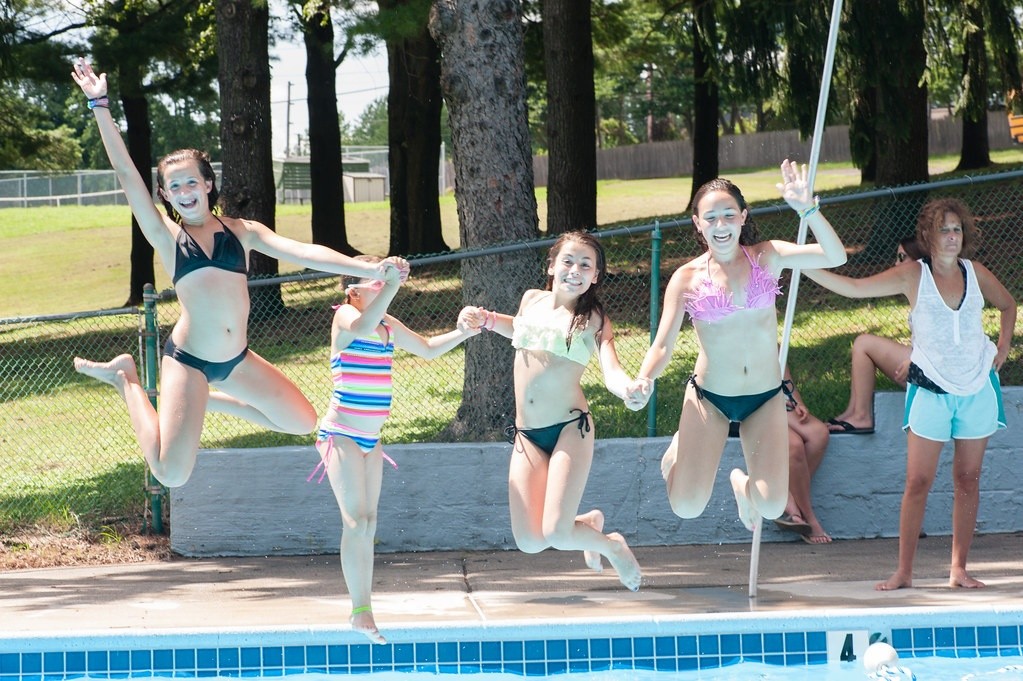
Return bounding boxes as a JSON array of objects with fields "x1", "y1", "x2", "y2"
[
  {"x1": 822, "y1": 237, "x2": 925, "y2": 435},
  {"x1": 456, "y1": 233, "x2": 655, "y2": 595},
  {"x1": 315, "y1": 255, "x2": 489, "y2": 656},
  {"x1": 622, "y1": 157, "x2": 849, "y2": 534},
  {"x1": 67, "y1": 53, "x2": 410, "y2": 488},
  {"x1": 796, "y1": 196, "x2": 1017, "y2": 592},
  {"x1": 773, "y1": 337, "x2": 833, "y2": 545}
]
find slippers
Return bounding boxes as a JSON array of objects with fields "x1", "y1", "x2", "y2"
[
  {"x1": 772, "y1": 514, "x2": 813, "y2": 535},
  {"x1": 824, "y1": 419, "x2": 876, "y2": 433},
  {"x1": 797, "y1": 532, "x2": 833, "y2": 545}
]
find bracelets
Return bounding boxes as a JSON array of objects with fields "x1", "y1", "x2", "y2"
[
  {"x1": 87, "y1": 96, "x2": 109, "y2": 110},
  {"x1": 479, "y1": 308, "x2": 497, "y2": 331},
  {"x1": 797, "y1": 203, "x2": 820, "y2": 219}
]
